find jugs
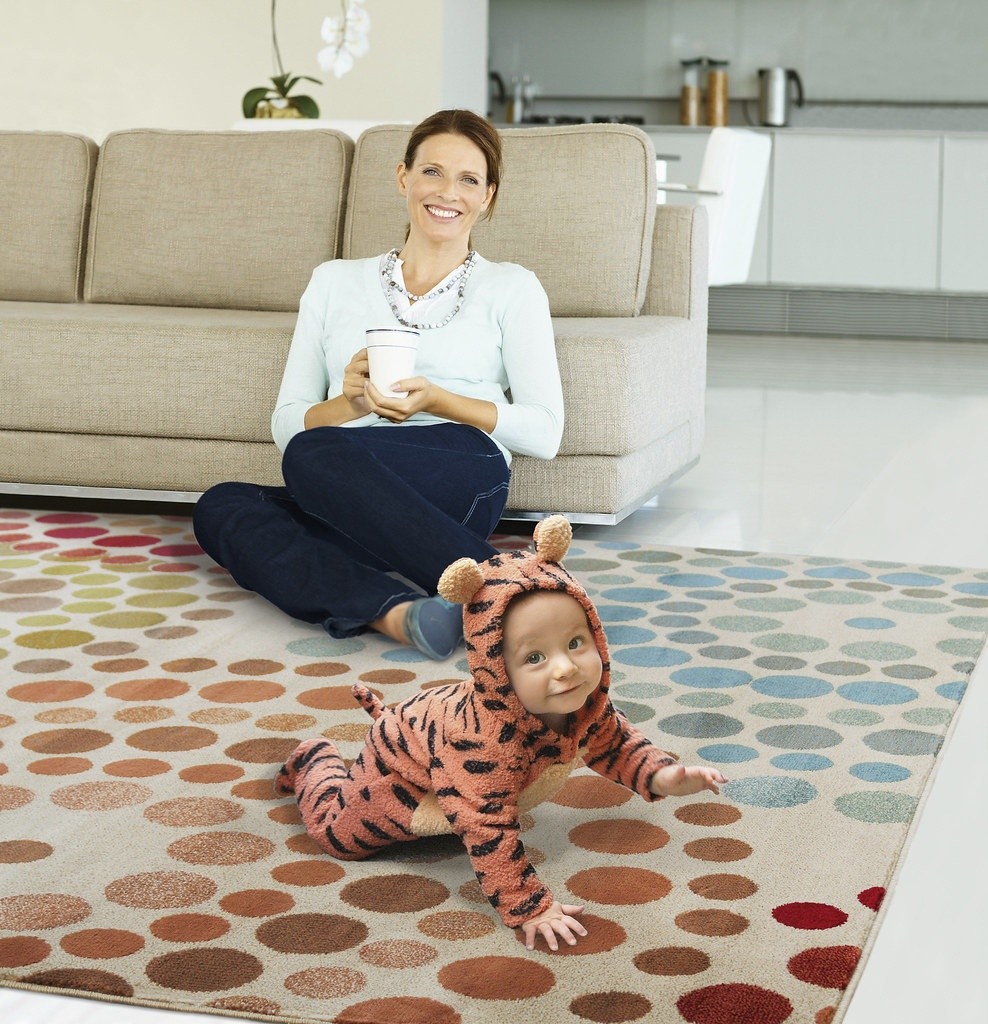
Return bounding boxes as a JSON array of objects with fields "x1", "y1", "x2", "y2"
[{"x1": 757, "y1": 67, "x2": 805, "y2": 126}]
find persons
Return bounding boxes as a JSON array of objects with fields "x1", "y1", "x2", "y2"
[
  {"x1": 190, "y1": 109, "x2": 565, "y2": 656},
  {"x1": 277, "y1": 515, "x2": 730, "y2": 951}
]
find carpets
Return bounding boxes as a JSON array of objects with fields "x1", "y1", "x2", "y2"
[{"x1": 0, "y1": 505, "x2": 987, "y2": 1024}]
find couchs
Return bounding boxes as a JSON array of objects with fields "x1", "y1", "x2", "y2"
[{"x1": 0, "y1": 122, "x2": 710, "y2": 536}]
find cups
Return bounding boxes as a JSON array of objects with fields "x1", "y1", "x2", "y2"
[
  {"x1": 363, "y1": 328, "x2": 420, "y2": 400},
  {"x1": 507, "y1": 73, "x2": 534, "y2": 125}
]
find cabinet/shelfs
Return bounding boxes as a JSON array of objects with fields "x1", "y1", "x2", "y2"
[{"x1": 648, "y1": 132, "x2": 988, "y2": 343}]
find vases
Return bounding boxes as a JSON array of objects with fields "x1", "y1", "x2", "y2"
[{"x1": 259, "y1": 101, "x2": 301, "y2": 120}]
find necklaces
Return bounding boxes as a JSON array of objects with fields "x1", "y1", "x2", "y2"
[{"x1": 383, "y1": 247, "x2": 473, "y2": 328}]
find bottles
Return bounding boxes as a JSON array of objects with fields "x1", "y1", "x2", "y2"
[
  {"x1": 680, "y1": 56, "x2": 703, "y2": 125},
  {"x1": 707, "y1": 56, "x2": 729, "y2": 125}
]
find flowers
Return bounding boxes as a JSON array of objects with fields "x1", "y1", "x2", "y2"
[{"x1": 243, "y1": 0, "x2": 371, "y2": 120}]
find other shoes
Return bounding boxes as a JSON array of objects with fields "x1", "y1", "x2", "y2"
[{"x1": 405, "y1": 592, "x2": 466, "y2": 659}]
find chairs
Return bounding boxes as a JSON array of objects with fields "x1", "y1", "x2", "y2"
[{"x1": 699, "y1": 127, "x2": 770, "y2": 288}]
